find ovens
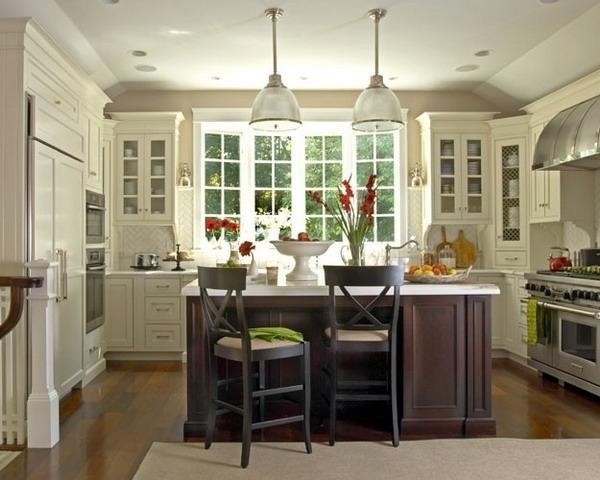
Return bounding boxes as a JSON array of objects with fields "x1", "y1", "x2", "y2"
[
  {"x1": 520, "y1": 295, "x2": 600, "y2": 397},
  {"x1": 85, "y1": 248, "x2": 107, "y2": 335}
]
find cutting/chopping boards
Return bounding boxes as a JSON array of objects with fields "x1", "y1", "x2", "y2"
[{"x1": 436, "y1": 226, "x2": 479, "y2": 266}]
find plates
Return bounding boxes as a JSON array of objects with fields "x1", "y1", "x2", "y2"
[
  {"x1": 469, "y1": 183, "x2": 480, "y2": 195},
  {"x1": 403, "y1": 267, "x2": 472, "y2": 283},
  {"x1": 441, "y1": 144, "x2": 481, "y2": 156},
  {"x1": 152, "y1": 165, "x2": 164, "y2": 176},
  {"x1": 125, "y1": 177, "x2": 137, "y2": 215}
]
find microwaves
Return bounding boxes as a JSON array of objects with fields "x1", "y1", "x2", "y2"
[{"x1": 87, "y1": 203, "x2": 106, "y2": 244}]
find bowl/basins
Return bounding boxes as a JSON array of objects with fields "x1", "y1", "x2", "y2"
[
  {"x1": 468, "y1": 161, "x2": 481, "y2": 175},
  {"x1": 442, "y1": 184, "x2": 451, "y2": 194},
  {"x1": 137, "y1": 254, "x2": 159, "y2": 267}
]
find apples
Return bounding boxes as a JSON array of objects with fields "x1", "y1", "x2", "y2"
[
  {"x1": 282, "y1": 232, "x2": 320, "y2": 241},
  {"x1": 408, "y1": 263, "x2": 457, "y2": 277}
]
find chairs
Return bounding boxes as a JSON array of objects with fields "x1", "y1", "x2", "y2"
[
  {"x1": 325, "y1": 267, "x2": 408, "y2": 449},
  {"x1": 195, "y1": 262, "x2": 315, "y2": 468}
]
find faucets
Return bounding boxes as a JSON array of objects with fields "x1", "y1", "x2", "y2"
[{"x1": 384, "y1": 240, "x2": 420, "y2": 266}]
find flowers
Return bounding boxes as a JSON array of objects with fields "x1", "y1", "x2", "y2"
[
  {"x1": 237, "y1": 239, "x2": 255, "y2": 261},
  {"x1": 203, "y1": 215, "x2": 241, "y2": 245},
  {"x1": 305, "y1": 174, "x2": 385, "y2": 246}
]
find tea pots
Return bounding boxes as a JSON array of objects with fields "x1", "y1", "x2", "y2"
[{"x1": 544, "y1": 245, "x2": 572, "y2": 272}]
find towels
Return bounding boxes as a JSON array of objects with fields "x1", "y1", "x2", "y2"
[
  {"x1": 527, "y1": 299, "x2": 546, "y2": 346},
  {"x1": 248, "y1": 325, "x2": 305, "y2": 345}
]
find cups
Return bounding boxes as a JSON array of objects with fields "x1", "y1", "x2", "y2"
[
  {"x1": 266, "y1": 265, "x2": 279, "y2": 283},
  {"x1": 124, "y1": 148, "x2": 136, "y2": 157}
]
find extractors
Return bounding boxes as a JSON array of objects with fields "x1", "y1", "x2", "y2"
[{"x1": 531, "y1": 96, "x2": 600, "y2": 173}]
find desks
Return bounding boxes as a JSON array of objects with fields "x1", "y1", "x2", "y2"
[{"x1": 179, "y1": 276, "x2": 495, "y2": 441}]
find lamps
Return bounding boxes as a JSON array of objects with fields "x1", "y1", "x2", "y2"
[
  {"x1": 177, "y1": 162, "x2": 192, "y2": 190},
  {"x1": 249, "y1": 6, "x2": 303, "y2": 134},
  {"x1": 352, "y1": 10, "x2": 405, "y2": 135},
  {"x1": 406, "y1": 160, "x2": 425, "y2": 190}
]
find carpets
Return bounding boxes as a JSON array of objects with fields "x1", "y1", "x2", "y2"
[{"x1": 130, "y1": 438, "x2": 600, "y2": 480}]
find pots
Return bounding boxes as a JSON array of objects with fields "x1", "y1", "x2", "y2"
[{"x1": 575, "y1": 247, "x2": 600, "y2": 269}]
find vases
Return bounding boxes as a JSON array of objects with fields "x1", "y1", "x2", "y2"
[
  {"x1": 213, "y1": 246, "x2": 236, "y2": 266},
  {"x1": 246, "y1": 258, "x2": 259, "y2": 277},
  {"x1": 340, "y1": 247, "x2": 367, "y2": 267}
]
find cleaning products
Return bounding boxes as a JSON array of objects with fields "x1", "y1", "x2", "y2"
[{"x1": 438, "y1": 245, "x2": 457, "y2": 271}]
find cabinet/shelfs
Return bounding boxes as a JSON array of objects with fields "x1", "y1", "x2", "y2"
[
  {"x1": 104, "y1": 270, "x2": 140, "y2": 362},
  {"x1": 2, "y1": 17, "x2": 85, "y2": 451},
  {"x1": 487, "y1": 114, "x2": 530, "y2": 272},
  {"x1": 85, "y1": 73, "x2": 114, "y2": 195},
  {"x1": 519, "y1": 88, "x2": 594, "y2": 226},
  {"x1": 417, "y1": 111, "x2": 499, "y2": 223},
  {"x1": 107, "y1": 110, "x2": 186, "y2": 256},
  {"x1": 140, "y1": 272, "x2": 184, "y2": 363},
  {"x1": 485, "y1": 274, "x2": 530, "y2": 358}
]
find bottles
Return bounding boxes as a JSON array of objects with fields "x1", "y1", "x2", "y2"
[{"x1": 440, "y1": 244, "x2": 457, "y2": 270}]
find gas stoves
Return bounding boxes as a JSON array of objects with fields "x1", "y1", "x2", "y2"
[{"x1": 522, "y1": 268, "x2": 600, "y2": 310}]
